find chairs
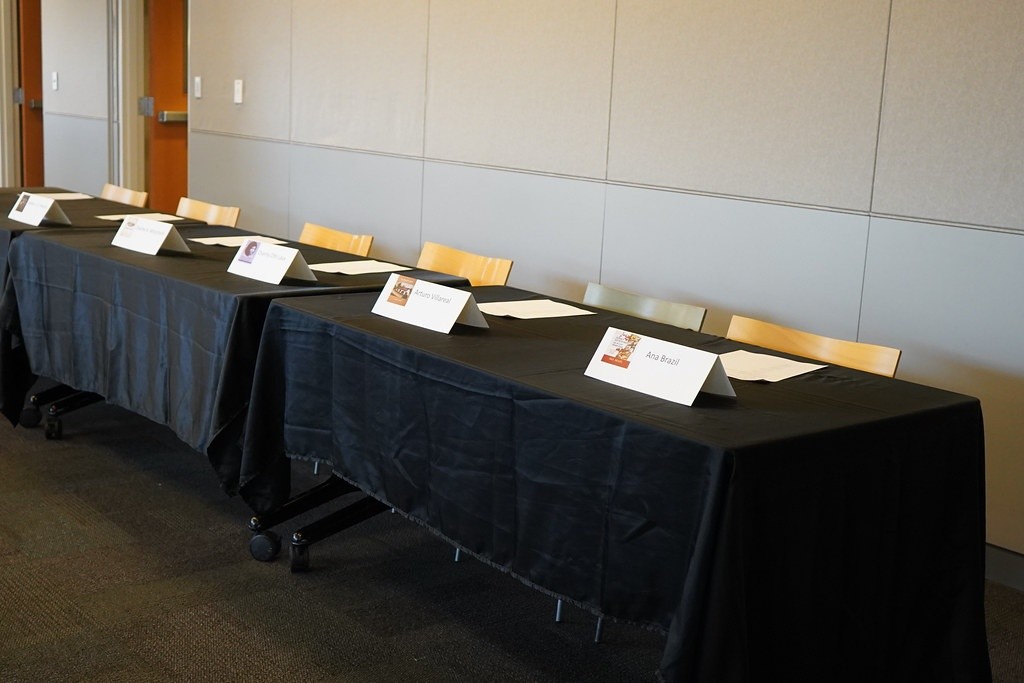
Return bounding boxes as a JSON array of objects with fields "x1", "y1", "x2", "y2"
[{"x1": 103, "y1": 184, "x2": 903, "y2": 377}]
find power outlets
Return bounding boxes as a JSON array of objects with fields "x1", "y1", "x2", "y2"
[{"x1": 194, "y1": 76, "x2": 202, "y2": 99}]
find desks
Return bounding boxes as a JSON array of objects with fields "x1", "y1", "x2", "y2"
[
  {"x1": 8, "y1": 224, "x2": 471, "y2": 535},
  {"x1": 238, "y1": 285, "x2": 992, "y2": 683},
  {"x1": 0, "y1": 187, "x2": 207, "y2": 429}
]
